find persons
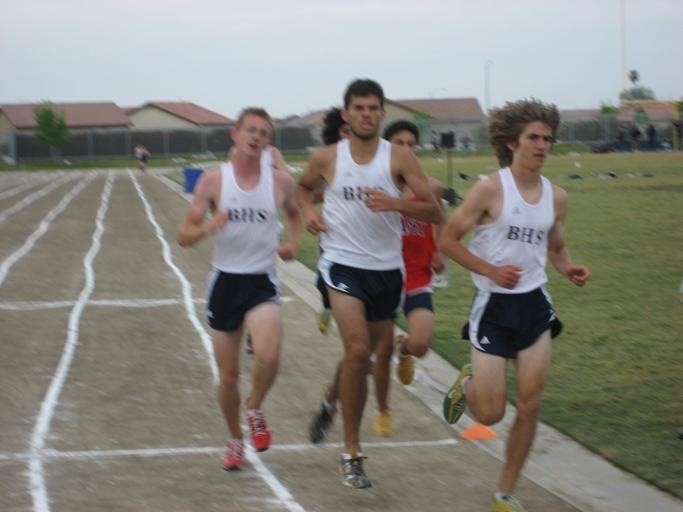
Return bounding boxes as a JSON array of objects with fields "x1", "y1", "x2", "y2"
[
  {"x1": 224, "y1": 142, "x2": 288, "y2": 353},
  {"x1": 137, "y1": 146, "x2": 150, "y2": 171},
  {"x1": 173, "y1": 107, "x2": 303, "y2": 473},
  {"x1": 372, "y1": 119, "x2": 444, "y2": 438},
  {"x1": 308, "y1": 105, "x2": 350, "y2": 338},
  {"x1": 132, "y1": 141, "x2": 144, "y2": 169},
  {"x1": 436, "y1": 95, "x2": 590, "y2": 512},
  {"x1": 292, "y1": 77, "x2": 445, "y2": 490}
]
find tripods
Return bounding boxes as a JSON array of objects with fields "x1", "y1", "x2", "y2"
[{"x1": 442, "y1": 149, "x2": 465, "y2": 204}]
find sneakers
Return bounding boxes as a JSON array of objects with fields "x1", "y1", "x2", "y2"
[
  {"x1": 221, "y1": 438, "x2": 244, "y2": 470},
  {"x1": 310, "y1": 398, "x2": 337, "y2": 443},
  {"x1": 319, "y1": 307, "x2": 332, "y2": 334},
  {"x1": 391, "y1": 335, "x2": 414, "y2": 386},
  {"x1": 245, "y1": 333, "x2": 255, "y2": 353},
  {"x1": 243, "y1": 397, "x2": 270, "y2": 451},
  {"x1": 443, "y1": 364, "x2": 473, "y2": 424},
  {"x1": 491, "y1": 491, "x2": 525, "y2": 512},
  {"x1": 339, "y1": 454, "x2": 371, "y2": 488},
  {"x1": 374, "y1": 409, "x2": 391, "y2": 436}
]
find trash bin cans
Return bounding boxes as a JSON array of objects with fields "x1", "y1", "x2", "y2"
[{"x1": 184, "y1": 168, "x2": 203, "y2": 192}]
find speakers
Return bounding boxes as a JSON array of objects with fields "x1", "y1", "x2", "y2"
[{"x1": 440, "y1": 132, "x2": 455, "y2": 148}]
